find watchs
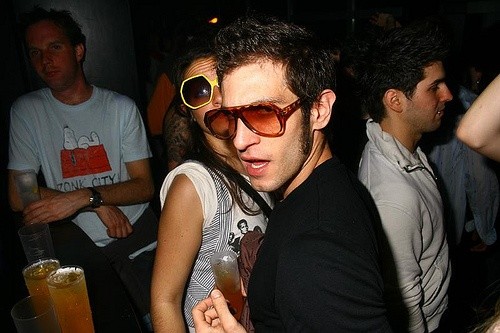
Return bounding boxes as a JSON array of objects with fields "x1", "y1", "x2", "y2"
[{"x1": 86, "y1": 186, "x2": 102, "y2": 209}]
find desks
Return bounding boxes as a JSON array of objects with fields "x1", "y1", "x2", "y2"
[{"x1": 0, "y1": 221, "x2": 144, "y2": 333}]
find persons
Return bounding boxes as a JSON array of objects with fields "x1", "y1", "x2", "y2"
[
  {"x1": 455, "y1": 67, "x2": 500, "y2": 172},
  {"x1": 148, "y1": 40, "x2": 271, "y2": 333},
  {"x1": 349, "y1": 22, "x2": 453, "y2": 333},
  {"x1": 6, "y1": 10, "x2": 161, "y2": 324},
  {"x1": 300, "y1": 12, "x2": 500, "y2": 305},
  {"x1": 192, "y1": 16, "x2": 409, "y2": 333}
]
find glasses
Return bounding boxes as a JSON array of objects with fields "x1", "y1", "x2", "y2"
[
  {"x1": 204, "y1": 99, "x2": 304, "y2": 140},
  {"x1": 180, "y1": 74, "x2": 221, "y2": 109}
]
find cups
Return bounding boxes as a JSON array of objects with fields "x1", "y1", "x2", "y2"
[
  {"x1": 13, "y1": 169, "x2": 40, "y2": 206},
  {"x1": 209, "y1": 251, "x2": 242, "y2": 321},
  {"x1": 45, "y1": 265, "x2": 95, "y2": 333},
  {"x1": 18, "y1": 223, "x2": 54, "y2": 264},
  {"x1": 22, "y1": 259, "x2": 60, "y2": 311},
  {"x1": 11, "y1": 294, "x2": 60, "y2": 333}
]
